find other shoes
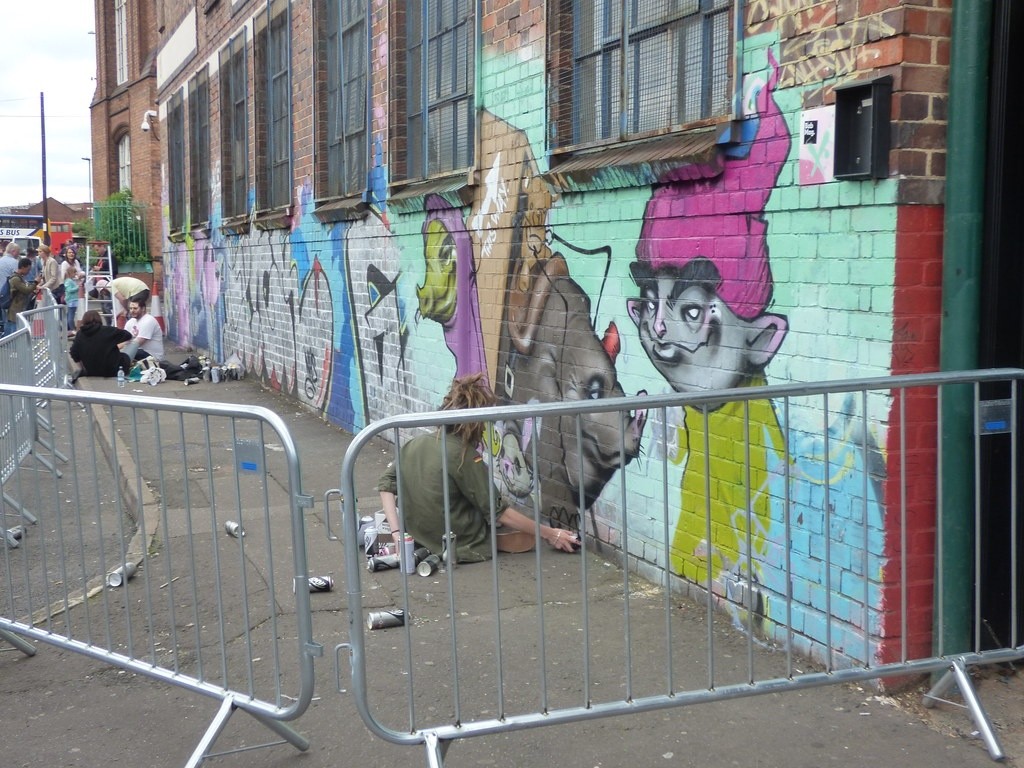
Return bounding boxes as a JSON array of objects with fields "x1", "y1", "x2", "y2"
[{"x1": 497, "y1": 531, "x2": 536, "y2": 552}]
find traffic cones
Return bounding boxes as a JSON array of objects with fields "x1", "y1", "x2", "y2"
[
  {"x1": 151, "y1": 282, "x2": 165, "y2": 337},
  {"x1": 31, "y1": 291, "x2": 45, "y2": 338}
]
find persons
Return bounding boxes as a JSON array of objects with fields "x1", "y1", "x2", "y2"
[
  {"x1": 0, "y1": 242, "x2": 117, "y2": 336},
  {"x1": 118, "y1": 299, "x2": 165, "y2": 361},
  {"x1": 97, "y1": 276, "x2": 151, "y2": 317},
  {"x1": 69, "y1": 309, "x2": 131, "y2": 381},
  {"x1": 377, "y1": 373, "x2": 582, "y2": 562}
]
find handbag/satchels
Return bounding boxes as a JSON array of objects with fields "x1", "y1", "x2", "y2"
[{"x1": 86, "y1": 268, "x2": 98, "y2": 299}]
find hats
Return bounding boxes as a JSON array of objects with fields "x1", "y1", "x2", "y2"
[{"x1": 95, "y1": 279, "x2": 108, "y2": 294}]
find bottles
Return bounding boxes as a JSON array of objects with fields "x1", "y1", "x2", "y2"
[
  {"x1": 548, "y1": 535, "x2": 581, "y2": 549},
  {"x1": 442, "y1": 532, "x2": 457, "y2": 570},
  {"x1": 108, "y1": 562, "x2": 136, "y2": 586},
  {"x1": 359, "y1": 515, "x2": 375, "y2": 551},
  {"x1": 7, "y1": 524, "x2": 27, "y2": 539},
  {"x1": 364, "y1": 526, "x2": 380, "y2": 561},
  {"x1": 367, "y1": 609, "x2": 411, "y2": 629},
  {"x1": 184, "y1": 377, "x2": 200, "y2": 385},
  {"x1": 292, "y1": 576, "x2": 335, "y2": 593},
  {"x1": 417, "y1": 554, "x2": 440, "y2": 576},
  {"x1": 367, "y1": 554, "x2": 400, "y2": 572},
  {"x1": 117, "y1": 366, "x2": 125, "y2": 387},
  {"x1": 399, "y1": 533, "x2": 415, "y2": 575},
  {"x1": 224, "y1": 520, "x2": 245, "y2": 537},
  {"x1": 202, "y1": 358, "x2": 245, "y2": 384},
  {"x1": 415, "y1": 547, "x2": 430, "y2": 566}
]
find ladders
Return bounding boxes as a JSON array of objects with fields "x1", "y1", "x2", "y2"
[{"x1": 86, "y1": 240, "x2": 117, "y2": 327}]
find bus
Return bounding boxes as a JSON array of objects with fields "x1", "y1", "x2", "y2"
[
  {"x1": 51, "y1": 222, "x2": 73, "y2": 256},
  {"x1": 0, "y1": 214, "x2": 51, "y2": 277}
]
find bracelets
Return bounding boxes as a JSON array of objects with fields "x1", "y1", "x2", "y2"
[
  {"x1": 391, "y1": 529, "x2": 400, "y2": 535},
  {"x1": 553, "y1": 529, "x2": 561, "y2": 545}
]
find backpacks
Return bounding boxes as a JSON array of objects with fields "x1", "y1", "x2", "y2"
[{"x1": 0, "y1": 275, "x2": 23, "y2": 309}]
